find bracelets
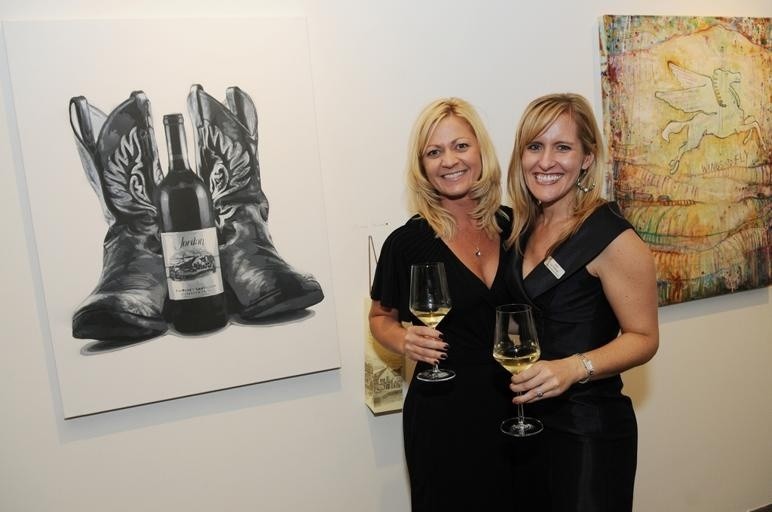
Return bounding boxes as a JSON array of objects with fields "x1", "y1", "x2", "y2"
[{"x1": 575, "y1": 352, "x2": 595, "y2": 385}]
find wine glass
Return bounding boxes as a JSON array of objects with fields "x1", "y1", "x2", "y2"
[
  {"x1": 493, "y1": 304, "x2": 545, "y2": 437},
  {"x1": 409, "y1": 263, "x2": 456, "y2": 382}
]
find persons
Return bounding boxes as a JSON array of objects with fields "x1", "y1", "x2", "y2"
[
  {"x1": 367, "y1": 94, "x2": 565, "y2": 511},
  {"x1": 502, "y1": 91, "x2": 661, "y2": 510}
]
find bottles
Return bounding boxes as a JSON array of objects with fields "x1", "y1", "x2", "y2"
[{"x1": 155, "y1": 113, "x2": 231, "y2": 336}]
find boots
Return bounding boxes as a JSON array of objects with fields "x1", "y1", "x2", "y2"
[
  {"x1": 182, "y1": 80, "x2": 329, "y2": 328},
  {"x1": 63, "y1": 88, "x2": 180, "y2": 346}
]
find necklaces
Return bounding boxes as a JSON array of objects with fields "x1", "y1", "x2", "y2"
[{"x1": 454, "y1": 218, "x2": 482, "y2": 257}]
